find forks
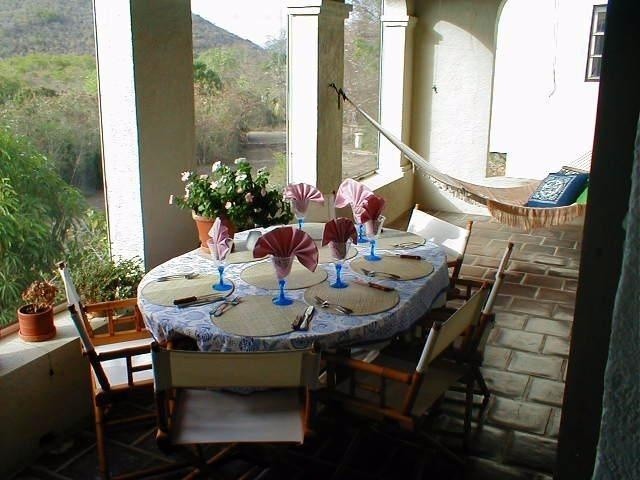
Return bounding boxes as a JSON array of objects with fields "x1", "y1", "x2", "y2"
[
  {"x1": 315, "y1": 296, "x2": 353, "y2": 314},
  {"x1": 361, "y1": 268, "x2": 401, "y2": 279}
]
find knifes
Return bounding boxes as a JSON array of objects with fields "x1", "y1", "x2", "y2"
[
  {"x1": 299, "y1": 304, "x2": 313, "y2": 330},
  {"x1": 174, "y1": 293, "x2": 224, "y2": 306},
  {"x1": 382, "y1": 254, "x2": 421, "y2": 261}
]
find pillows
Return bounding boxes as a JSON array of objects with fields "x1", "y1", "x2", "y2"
[
  {"x1": 527, "y1": 171, "x2": 590, "y2": 208},
  {"x1": 576, "y1": 174, "x2": 591, "y2": 205}
]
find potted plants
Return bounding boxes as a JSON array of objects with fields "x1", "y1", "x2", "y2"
[
  {"x1": 62, "y1": 209, "x2": 113, "y2": 318},
  {"x1": 17, "y1": 280, "x2": 59, "y2": 342},
  {"x1": 114, "y1": 256, "x2": 145, "y2": 315}
]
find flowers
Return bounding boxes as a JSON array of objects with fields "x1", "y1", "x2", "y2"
[{"x1": 170, "y1": 157, "x2": 294, "y2": 230}]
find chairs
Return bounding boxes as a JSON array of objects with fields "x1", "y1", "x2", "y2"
[
  {"x1": 322, "y1": 283, "x2": 492, "y2": 480},
  {"x1": 406, "y1": 204, "x2": 474, "y2": 289},
  {"x1": 59, "y1": 262, "x2": 200, "y2": 480},
  {"x1": 152, "y1": 342, "x2": 322, "y2": 480},
  {"x1": 392, "y1": 242, "x2": 514, "y2": 446}
]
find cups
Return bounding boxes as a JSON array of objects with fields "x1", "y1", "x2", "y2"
[
  {"x1": 292, "y1": 198, "x2": 310, "y2": 231},
  {"x1": 206, "y1": 238, "x2": 234, "y2": 291},
  {"x1": 364, "y1": 214, "x2": 385, "y2": 262},
  {"x1": 329, "y1": 238, "x2": 351, "y2": 289},
  {"x1": 272, "y1": 254, "x2": 294, "y2": 306},
  {"x1": 351, "y1": 206, "x2": 368, "y2": 243}
]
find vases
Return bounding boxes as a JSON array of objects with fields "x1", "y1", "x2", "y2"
[{"x1": 192, "y1": 211, "x2": 238, "y2": 253}]
